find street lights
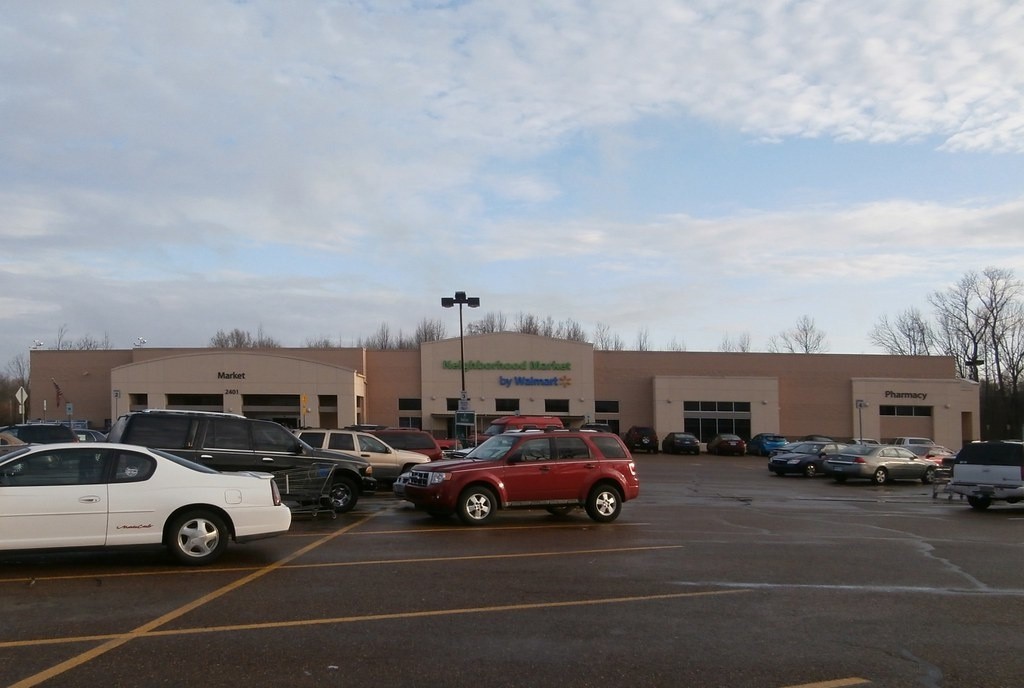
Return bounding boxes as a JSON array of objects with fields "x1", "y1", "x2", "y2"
[{"x1": 441, "y1": 290, "x2": 479, "y2": 391}]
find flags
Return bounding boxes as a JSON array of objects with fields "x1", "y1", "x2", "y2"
[{"x1": 53, "y1": 380, "x2": 65, "y2": 408}]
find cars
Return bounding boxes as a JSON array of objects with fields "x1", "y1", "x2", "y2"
[
  {"x1": 796, "y1": 434, "x2": 833, "y2": 442},
  {"x1": 904, "y1": 443, "x2": 956, "y2": 468},
  {"x1": 768, "y1": 441, "x2": 860, "y2": 479},
  {"x1": 846, "y1": 437, "x2": 881, "y2": 446},
  {"x1": 0, "y1": 442, "x2": 292, "y2": 567},
  {"x1": 707, "y1": 434, "x2": 747, "y2": 457},
  {"x1": 662, "y1": 432, "x2": 700, "y2": 456},
  {"x1": 747, "y1": 433, "x2": 790, "y2": 456},
  {"x1": 822, "y1": 443, "x2": 940, "y2": 486},
  {"x1": 453, "y1": 427, "x2": 596, "y2": 460},
  {"x1": 0, "y1": 420, "x2": 109, "y2": 478}
]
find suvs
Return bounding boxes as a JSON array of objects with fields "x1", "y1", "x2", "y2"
[
  {"x1": 290, "y1": 426, "x2": 432, "y2": 486},
  {"x1": 946, "y1": 440, "x2": 1024, "y2": 512},
  {"x1": 623, "y1": 425, "x2": 659, "y2": 454},
  {"x1": 887, "y1": 436, "x2": 935, "y2": 445},
  {"x1": 344, "y1": 423, "x2": 465, "y2": 463},
  {"x1": 108, "y1": 409, "x2": 378, "y2": 514},
  {"x1": 403, "y1": 427, "x2": 639, "y2": 526},
  {"x1": 581, "y1": 422, "x2": 613, "y2": 433},
  {"x1": 466, "y1": 414, "x2": 564, "y2": 447}
]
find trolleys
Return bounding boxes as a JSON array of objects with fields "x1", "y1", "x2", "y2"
[{"x1": 933, "y1": 468, "x2": 964, "y2": 501}]
